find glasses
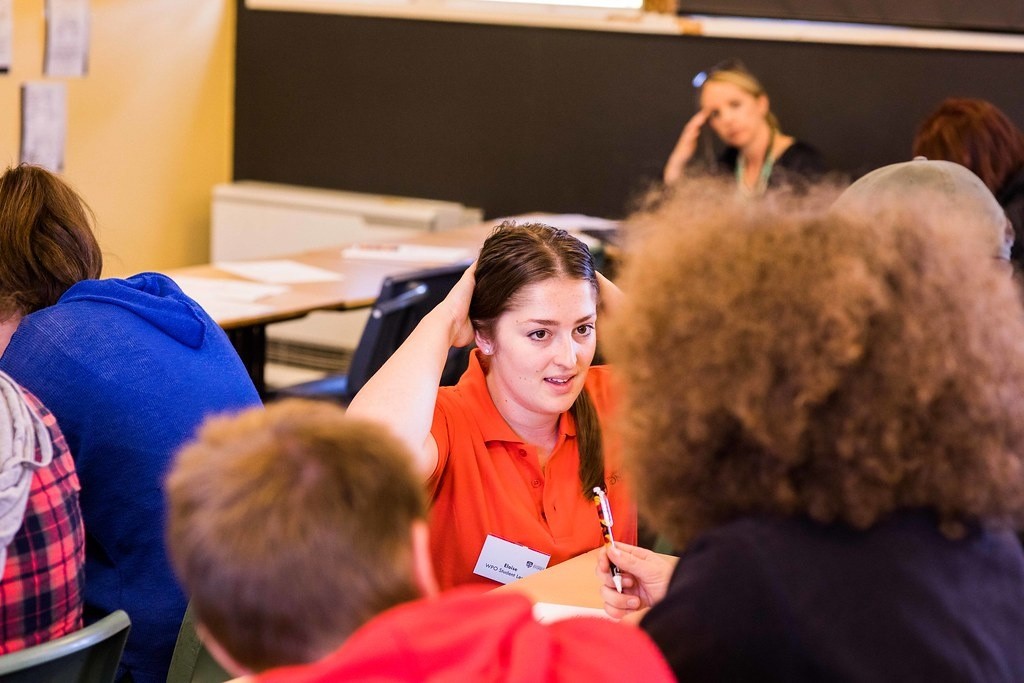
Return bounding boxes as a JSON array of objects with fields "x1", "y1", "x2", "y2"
[{"x1": 692, "y1": 57, "x2": 738, "y2": 88}]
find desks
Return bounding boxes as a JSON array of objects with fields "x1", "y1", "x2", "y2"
[
  {"x1": 220, "y1": 211, "x2": 622, "y2": 310},
  {"x1": 490, "y1": 539, "x2": 677, "y2": 625},
  {"x1": 160, "y1": 267, "x2": 343, "y2": 396}
]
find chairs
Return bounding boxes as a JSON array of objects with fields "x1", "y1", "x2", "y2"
[
  {"x1": 377, "y1": 260, "x2": 480, "y2": 386},
  {"x1": 0, "y1": 606, "x2": 134, "y2": 683},
  {"x1": 271, "y1": 284, "x2": 428, "y2": 408}
]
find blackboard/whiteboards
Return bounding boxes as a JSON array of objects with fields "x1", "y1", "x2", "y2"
[{"x1": 227, "y1": 1, "x2": 1022, "y2": 276}]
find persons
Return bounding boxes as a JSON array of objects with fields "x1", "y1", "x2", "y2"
[
  {"x1": 171, "y1": 402, "x2": 678, "y2": 683},
  {"x1": 596, "y1": 58, "x2": 1024, "y2": 683},
  {"x1": 0, "y1": 165, "x2": 264, "y2": 683},
  {"x1": 340, "y1": 220, "x2": 642, "y2": 592}
]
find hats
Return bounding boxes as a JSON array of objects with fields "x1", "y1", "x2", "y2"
[{"x1": 832, "y1": 155, "x2": 1015, "y2": 260}]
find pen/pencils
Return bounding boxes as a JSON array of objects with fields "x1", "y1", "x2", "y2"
[{"x1": 593, "y1": 485, "x2": 622, "y2": 593}]
